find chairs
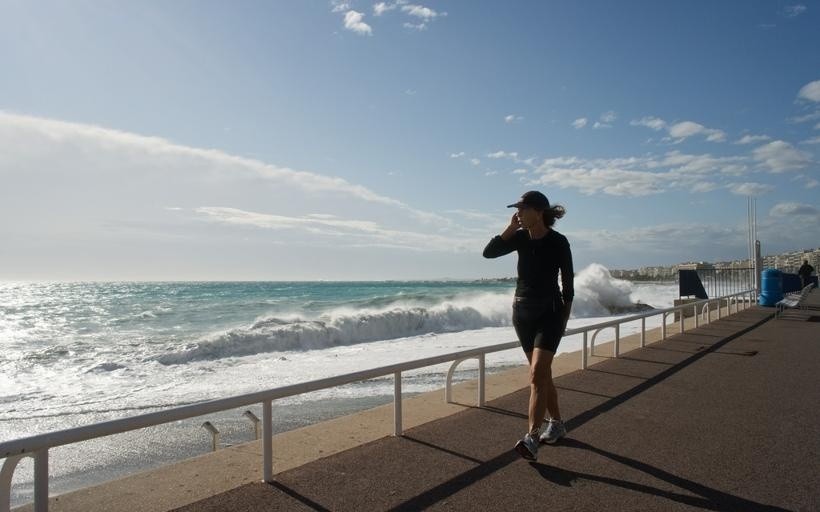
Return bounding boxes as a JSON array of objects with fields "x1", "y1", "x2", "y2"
[{"x1": 774, "y1": 282, "x2": 814, "y2": 320}]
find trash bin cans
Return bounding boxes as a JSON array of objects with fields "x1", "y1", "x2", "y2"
[{"x1": 758, "y1": 269, "x2": 784, "y2": 307}]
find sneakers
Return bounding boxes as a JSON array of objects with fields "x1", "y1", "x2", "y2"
[
  {"x1": 514, "y1": 433, "x2": 537, "y2": 463},
  {"x1": 539, "y1": 419, "x2": 567, "y2": 444}
]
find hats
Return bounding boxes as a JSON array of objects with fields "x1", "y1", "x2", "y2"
[{"x1": 506, "y1": 191, "x2": 550, "y2": 210}]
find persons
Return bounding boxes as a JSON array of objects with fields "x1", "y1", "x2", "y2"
[
  {"x1": 798, "y1": 260, "x2": 815, "y2": 292},
  {"x1": 482, "y1": 191, "x2": 574, "y2": 463}
]
